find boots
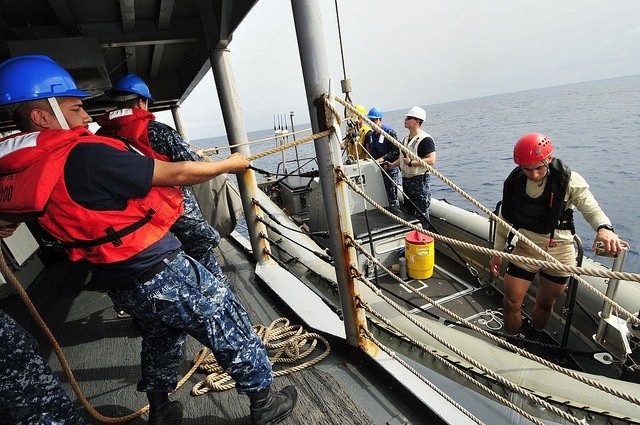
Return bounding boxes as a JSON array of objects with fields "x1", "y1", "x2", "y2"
[
  {"x1": 147, "y1": 391, "x2": 184, "y2": 424},
  {"x1": 248, "y1": 385, "x2": 297, "y2": 425}
]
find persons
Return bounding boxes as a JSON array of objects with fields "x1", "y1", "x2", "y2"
[
  {"x1": 0, "y1": 312, "x2": 88, "y2": 425},
  {"x1": 94, "y1": 74, "x2": 235, "y2": 296},
  {"x1": 0, "y1": 55, "x2": 298, "y2": 425},
  {"x1": 364, "y1": 107, "x2": 400, "y2": 210},
  {"x1": 490, "y1": 133, "x2": 631, "y2": 354},
  {"x1": 384, "y1": 106, "x2": 436, "y2": 231},
  {"x1": 341, "y1": 105, "x2": 372, "y2": 163}
]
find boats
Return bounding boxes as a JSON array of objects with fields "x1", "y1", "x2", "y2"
[{"x1": 256, "y1": 111, "x2": 640, "y2": 425}]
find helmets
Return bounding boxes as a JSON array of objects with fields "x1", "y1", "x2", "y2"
[
  {"x1": 355, "y1": 105, "x2": 367, "y2": 114},
  {"x1": 105, "y1": 74, "x2": 154, "y2": 105},
  {"x1": 406, "y1": 106, "x2": 426, "y2": 121},
  {"x1": 514, "y1": 133, "x2": 554, "y2": 164},
  {"x1": 0, "y1": 54, "x2": 91, "y2": 105},
  {"x1": 368, "y1": 107, "x2": 383, "y2": 118}
]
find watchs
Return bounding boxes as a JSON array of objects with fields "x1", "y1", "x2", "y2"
[
  {"x1": 597, "y1": 224, "x2": 613, "y2": 232},
  {"x1": 408, "y1": 160, "x2": 412, "y2": 167}
]
